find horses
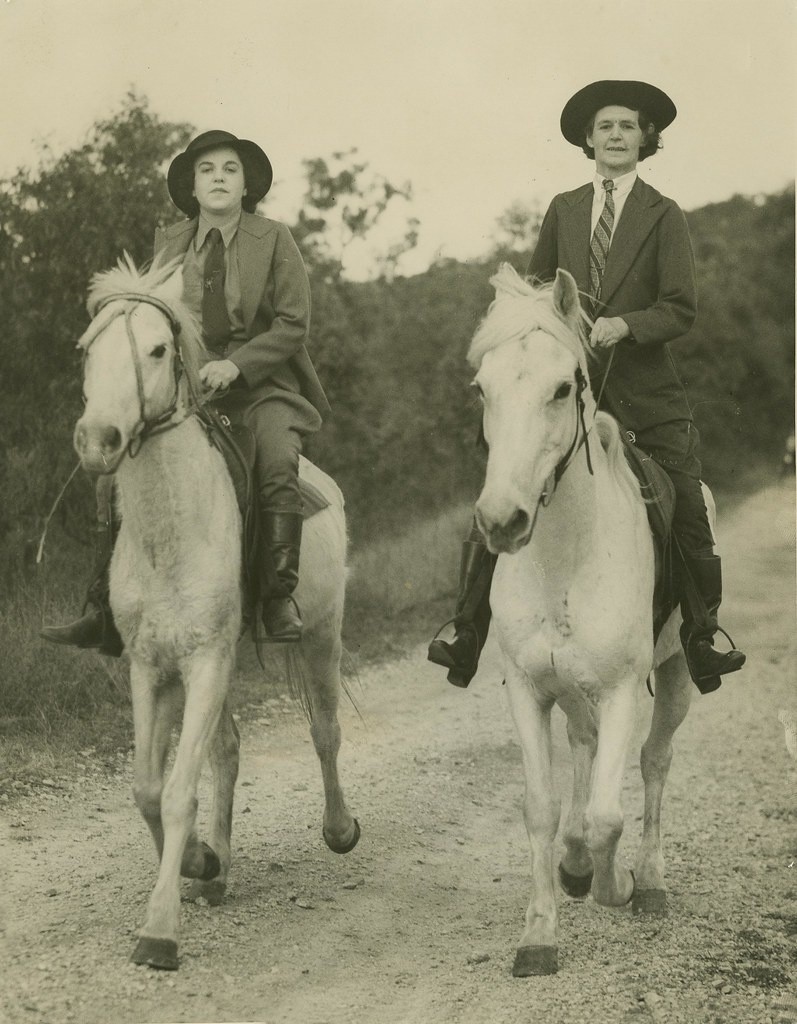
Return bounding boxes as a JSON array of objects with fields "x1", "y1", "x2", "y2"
[
  {"x1": 465, "y1": 260, "x2": 717, "y2": 976},
  {"x1": 71, "y1": 247, "x2": 360, "y2": 970}
]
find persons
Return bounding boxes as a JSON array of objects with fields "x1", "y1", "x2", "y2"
[
  {"x1": 426, "y1": 80, "x2": 744, "y2": 696},
  {"x1": 37, "y1": 130, "x2": 335, "y2": 660}
]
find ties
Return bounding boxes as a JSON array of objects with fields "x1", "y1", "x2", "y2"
[
  {"x1": 585, "y1": 179, "x2": 615, "y2": 316},
  {"x1": 201, "y1": 228, "x2": 232, "y2": 355}
]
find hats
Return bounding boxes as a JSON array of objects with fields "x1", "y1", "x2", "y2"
[
  {"x1": 560, "y1": 80, "x2": 677, "y2": 147},
  {"x1": 167, "y1": 130, "x2": 273, "y2": 215}
]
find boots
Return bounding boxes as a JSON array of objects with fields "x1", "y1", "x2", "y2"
[
  {"x1": 39, "y1": 530, "x2": 125, "y2": 658},
  {"x1": 260, "y1": 511, "x2": 305, "y2": 638},
  {"x1": 679, "y1": 555, "x2": 746, "y2": 694},
  {"x1": 428, "y1": 542, "x2": 499, "y2": 688}
]
